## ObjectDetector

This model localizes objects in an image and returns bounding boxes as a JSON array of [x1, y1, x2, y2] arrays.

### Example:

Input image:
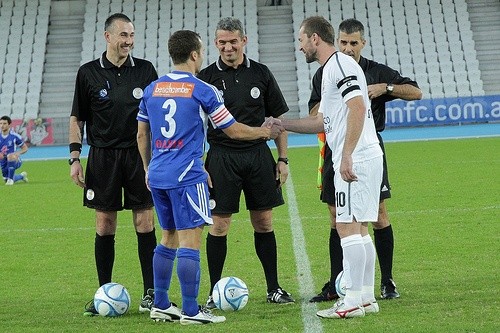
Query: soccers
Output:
[[213, 277, 249, 311], [94, 283, 130, 316]]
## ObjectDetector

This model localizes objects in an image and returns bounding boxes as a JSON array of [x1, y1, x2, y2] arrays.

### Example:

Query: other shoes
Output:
[[20, 171, 28, 183], [5, 177, 14, 185]]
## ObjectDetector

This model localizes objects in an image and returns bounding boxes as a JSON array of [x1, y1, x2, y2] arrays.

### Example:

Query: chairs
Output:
[[0, 0, 51, 119], [80, 0, 260, 78], [292, 0, 485, 118]]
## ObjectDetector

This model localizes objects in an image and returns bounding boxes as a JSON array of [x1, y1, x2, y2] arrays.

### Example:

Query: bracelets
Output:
[[69, 142, 82, 153]]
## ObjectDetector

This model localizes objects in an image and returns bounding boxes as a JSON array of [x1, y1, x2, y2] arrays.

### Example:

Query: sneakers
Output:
[[149, 301, 182, 322], [316, 299, 366, 319], [138, 288, 155, 312], [308, 281, 340, 302], [265, 287, 296, 305], [205, 293, 218, 311], [84, 298, 100, 316], [379, 278, 401, 299], [363, 298, 380, 313], [180, 304, 226, 325]]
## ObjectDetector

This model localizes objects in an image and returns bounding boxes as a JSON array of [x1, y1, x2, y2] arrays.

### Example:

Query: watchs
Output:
[[278, 157, 288, 165], [69, 158, 81, 165], [386, 83, 394, 95]]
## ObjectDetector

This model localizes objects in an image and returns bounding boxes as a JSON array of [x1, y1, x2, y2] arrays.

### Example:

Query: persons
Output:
[[0, 116, 28, 185], [261, 16, 383, 318], [69, 13, 177, 316], [307, 20, 422, 303], [136, 30, 286, 325], [195, 17, 295, 310]]
